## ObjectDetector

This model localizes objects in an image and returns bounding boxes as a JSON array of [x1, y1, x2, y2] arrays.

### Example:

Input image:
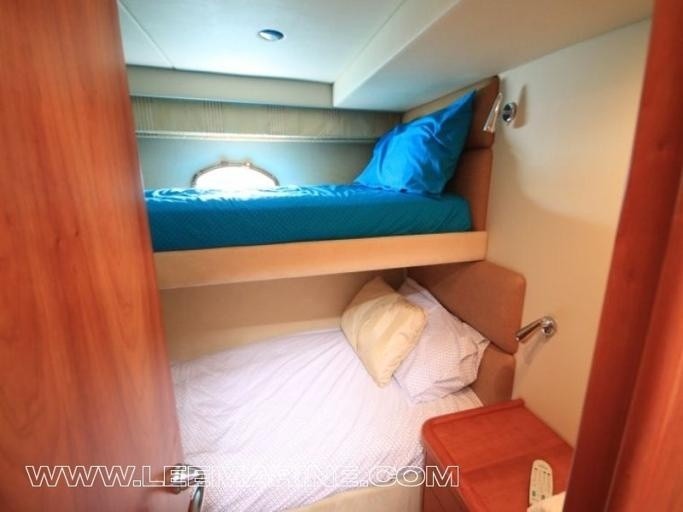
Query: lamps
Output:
[[481, 92, 518, 134]]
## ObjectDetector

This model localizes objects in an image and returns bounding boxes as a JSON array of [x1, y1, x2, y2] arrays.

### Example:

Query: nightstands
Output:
[[419, 398, 576, 512]]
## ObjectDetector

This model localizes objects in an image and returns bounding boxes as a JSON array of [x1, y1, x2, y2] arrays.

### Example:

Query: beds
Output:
[[166, 262, 526, 511], [141, 74, 501, 292]]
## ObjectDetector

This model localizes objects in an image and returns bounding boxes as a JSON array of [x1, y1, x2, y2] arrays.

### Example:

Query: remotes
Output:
[[528, 458, 553, 505]]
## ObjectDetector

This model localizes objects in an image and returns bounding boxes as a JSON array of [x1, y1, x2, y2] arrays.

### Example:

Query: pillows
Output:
[[340, 275, 491, 407], [354, 90, 475, 196]]
